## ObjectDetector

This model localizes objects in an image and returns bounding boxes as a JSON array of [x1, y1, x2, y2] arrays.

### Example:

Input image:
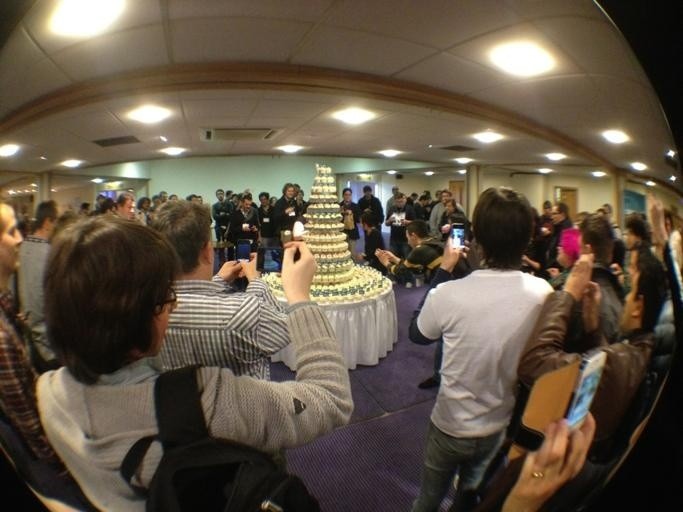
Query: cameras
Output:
[[257, 247, 301, 272]]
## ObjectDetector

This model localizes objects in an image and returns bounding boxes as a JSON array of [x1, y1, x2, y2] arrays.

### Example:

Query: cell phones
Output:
[[400, 218, 404, 222], [236, 239, 251, 263], [562, 350, 607, 432], [451, 223, 465, 249], [249, 223, 254, 229]]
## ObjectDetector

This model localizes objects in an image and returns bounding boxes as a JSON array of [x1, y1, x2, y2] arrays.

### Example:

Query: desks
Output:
[[262, 274, 398, 369]]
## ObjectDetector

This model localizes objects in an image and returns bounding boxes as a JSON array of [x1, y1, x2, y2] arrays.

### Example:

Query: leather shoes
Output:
[[418, 377, 438, 388]]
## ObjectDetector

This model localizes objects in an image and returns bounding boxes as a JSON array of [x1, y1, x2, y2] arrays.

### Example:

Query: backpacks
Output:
[[121, 364, 324, 512]]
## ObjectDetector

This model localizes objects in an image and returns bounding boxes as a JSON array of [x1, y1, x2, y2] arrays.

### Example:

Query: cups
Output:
[[261, 165, 390, 304]]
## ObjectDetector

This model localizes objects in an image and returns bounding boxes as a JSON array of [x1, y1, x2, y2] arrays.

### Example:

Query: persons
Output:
[[3, 181, 683, 512]]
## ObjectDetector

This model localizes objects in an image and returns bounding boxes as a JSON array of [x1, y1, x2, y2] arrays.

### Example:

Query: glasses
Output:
[[153, 287, 177, 315]]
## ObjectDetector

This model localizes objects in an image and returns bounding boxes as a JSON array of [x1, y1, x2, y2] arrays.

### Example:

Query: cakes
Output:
[[258, 162, 391, 306]]
